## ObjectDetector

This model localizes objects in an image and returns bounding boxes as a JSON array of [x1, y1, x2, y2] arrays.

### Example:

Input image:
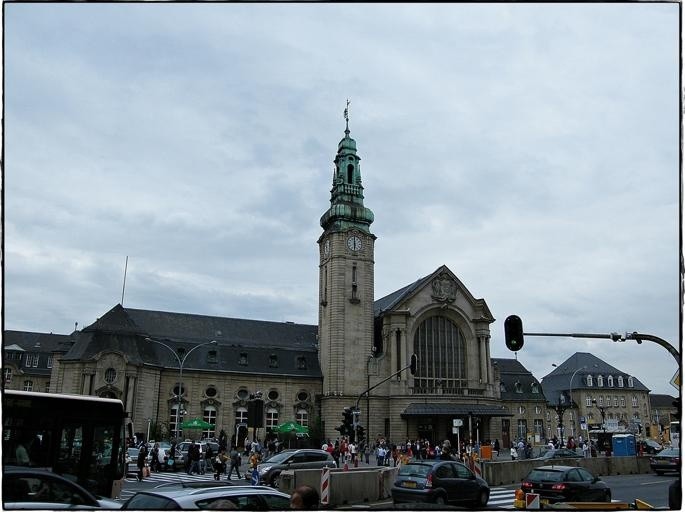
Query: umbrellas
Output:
[[178, 414, 217, 435], [271, 418, 311, 447]]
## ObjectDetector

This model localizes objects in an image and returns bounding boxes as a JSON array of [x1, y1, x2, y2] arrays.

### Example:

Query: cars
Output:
[[521, 465, 610, 505], [245, 450, 338, 486], [390, 459, 490, 509], [650, 448, 681, 476], [126, 441, 220, 480], [635, 437, 662, 454], [122, 481, 290, 510], [3, 468, 123, 509]]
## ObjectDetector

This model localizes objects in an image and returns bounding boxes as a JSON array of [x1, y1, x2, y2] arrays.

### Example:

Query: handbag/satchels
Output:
[[143, 465, 151, 478]]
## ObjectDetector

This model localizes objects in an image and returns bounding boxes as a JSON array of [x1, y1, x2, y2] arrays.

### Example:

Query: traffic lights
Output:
[[335, 407, 367, 444], [410, 354, 417, 374], [463, 414, 482, 429], [504, 315, 523, 351]]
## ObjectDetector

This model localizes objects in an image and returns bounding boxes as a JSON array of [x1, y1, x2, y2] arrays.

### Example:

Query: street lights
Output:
[[145, 336, 218, 441], [552, 364, 588, 438], [247, 391, 264, 443]]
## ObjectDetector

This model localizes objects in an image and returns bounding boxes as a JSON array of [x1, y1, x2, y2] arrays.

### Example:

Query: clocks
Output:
[[323, 239, 331, 256], [346, 233, 363, 252]]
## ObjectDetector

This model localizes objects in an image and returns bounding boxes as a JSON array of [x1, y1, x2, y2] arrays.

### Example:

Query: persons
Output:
[[583, 439, 589, 457], [321, 434, 582, 467], [290, 485, 320, 510], [124, 436, 287, 481], [15, 435, 35, 466]]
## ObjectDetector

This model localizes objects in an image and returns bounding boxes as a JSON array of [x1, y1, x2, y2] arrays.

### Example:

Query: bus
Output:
[[2, 385, 125, 498], [670, 421, 681, 447], [588, 429, 633, 454]]
